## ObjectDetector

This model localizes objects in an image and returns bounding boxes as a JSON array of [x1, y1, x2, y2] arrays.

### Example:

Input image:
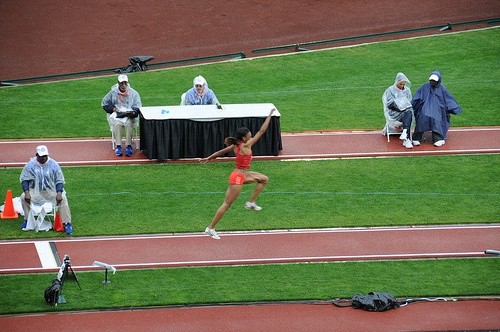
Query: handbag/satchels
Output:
[[44, 278, 60, 306]]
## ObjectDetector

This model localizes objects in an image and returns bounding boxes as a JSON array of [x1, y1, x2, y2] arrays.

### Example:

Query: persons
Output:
[[410, 70, 461, 146], [101, 74, 142, 156], [20, 145, 73, 235], [184, 76, 222, 109], [382, 72, 415, 149], [198, 108, 276, 240]]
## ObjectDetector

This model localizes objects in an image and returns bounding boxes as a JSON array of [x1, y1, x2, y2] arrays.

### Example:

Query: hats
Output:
[[194, 76, 204, 86], [36, 145, 49, 156], [118, 74, 128, 82], [428, 74, 439, 81]]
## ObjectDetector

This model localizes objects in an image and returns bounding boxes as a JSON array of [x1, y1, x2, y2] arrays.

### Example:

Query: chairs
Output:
[[384, 96, 413, 142], [112, 118, 139, 151], [27, 198, 57, 231]]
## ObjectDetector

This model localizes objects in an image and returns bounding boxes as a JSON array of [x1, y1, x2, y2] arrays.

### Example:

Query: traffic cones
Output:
[[0, 189, 18, 219]]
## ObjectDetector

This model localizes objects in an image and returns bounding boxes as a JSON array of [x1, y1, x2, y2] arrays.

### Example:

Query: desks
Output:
[[139, 102, 283, 159]]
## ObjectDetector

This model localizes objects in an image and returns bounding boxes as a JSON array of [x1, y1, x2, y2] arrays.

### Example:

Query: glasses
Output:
[[195, 85, 202, 87], [399, 83, 405, 85]]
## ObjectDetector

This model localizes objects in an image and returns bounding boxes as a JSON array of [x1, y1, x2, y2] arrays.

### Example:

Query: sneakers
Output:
[[399, 134, 407, 140], [403, 140, 413, 148], [21, 220, 27, 229], [64, 222, 73, 235], [412, 140, 421, 146], [114, 146, 123, 156], [205, 227, 220, 240], [125, 146, 133, 157], [433, 139, 445, 147], [245, 201, 262, 211]]
